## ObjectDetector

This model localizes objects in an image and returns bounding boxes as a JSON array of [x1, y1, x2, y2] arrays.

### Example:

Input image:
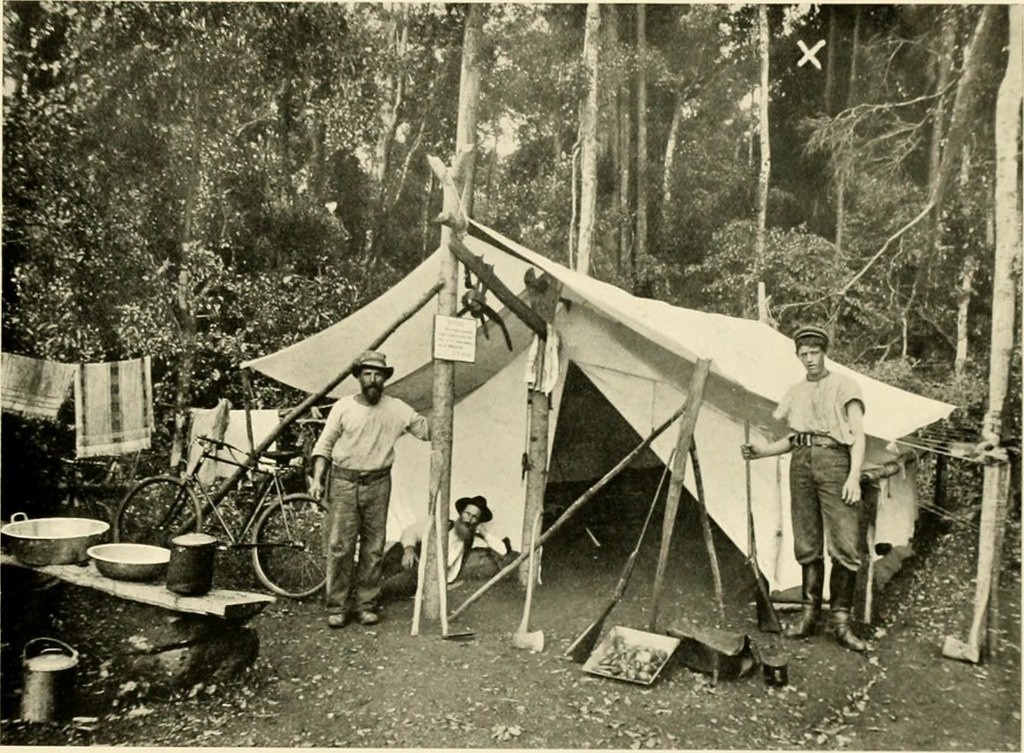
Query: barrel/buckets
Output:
[[21, 636, 80, 722], [166, 532, 218, 597]]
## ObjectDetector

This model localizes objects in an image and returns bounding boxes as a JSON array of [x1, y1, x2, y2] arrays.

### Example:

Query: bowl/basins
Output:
[[0, 511, 111, 566], [84, 541, 172, 583]]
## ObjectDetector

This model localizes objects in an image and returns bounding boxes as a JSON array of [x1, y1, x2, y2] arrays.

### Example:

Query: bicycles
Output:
[[112, 433, 343, 601]]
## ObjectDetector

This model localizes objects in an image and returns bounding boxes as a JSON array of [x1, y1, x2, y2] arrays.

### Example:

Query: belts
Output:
[[332, 469, 392, 483], [792, 433, 835, 446]]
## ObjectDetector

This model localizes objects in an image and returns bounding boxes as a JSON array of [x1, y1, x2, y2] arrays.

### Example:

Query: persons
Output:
[[741, 325, 870, 651], [308, 351, 432, 626], [375, 496, 510, 596]]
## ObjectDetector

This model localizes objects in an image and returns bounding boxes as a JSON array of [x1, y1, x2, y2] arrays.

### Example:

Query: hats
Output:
[[351, 351, 397, 378], [453, 495, 493, 524], [793, 324, 829, 341]]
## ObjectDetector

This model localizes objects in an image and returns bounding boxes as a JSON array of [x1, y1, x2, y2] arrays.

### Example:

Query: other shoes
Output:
[[355, 611, 379, 623], [328, 611, 349, 628]]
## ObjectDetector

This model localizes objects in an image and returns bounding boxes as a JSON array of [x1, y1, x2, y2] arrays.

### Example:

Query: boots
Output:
[[789, 565, 824, 637], [827, 565, 866, 654]]
